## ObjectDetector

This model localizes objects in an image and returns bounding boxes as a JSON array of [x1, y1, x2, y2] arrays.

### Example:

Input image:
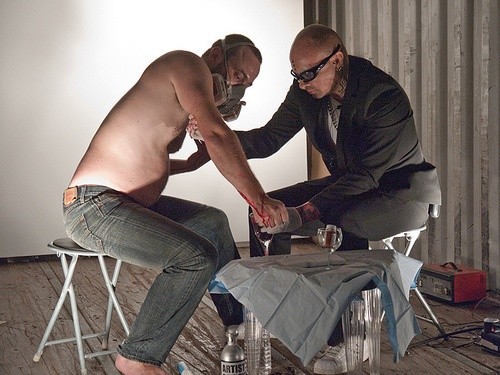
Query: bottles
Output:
[[220, 330, 247, 375]]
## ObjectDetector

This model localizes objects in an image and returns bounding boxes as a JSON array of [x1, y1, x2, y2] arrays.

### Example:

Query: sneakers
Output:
[[237, 322, 278, 339], [314, 332, 372, 374]]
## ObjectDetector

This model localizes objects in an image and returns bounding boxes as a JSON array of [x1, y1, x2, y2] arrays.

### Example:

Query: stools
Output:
[[368, 225, 450, 355], [32, 238, 131, 375]]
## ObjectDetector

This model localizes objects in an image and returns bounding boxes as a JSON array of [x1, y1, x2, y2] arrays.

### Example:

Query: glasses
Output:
[[290, 44, 340, 82]]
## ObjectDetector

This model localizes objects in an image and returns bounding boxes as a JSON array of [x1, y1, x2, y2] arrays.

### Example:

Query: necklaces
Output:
[[326, 96, 338, 130]]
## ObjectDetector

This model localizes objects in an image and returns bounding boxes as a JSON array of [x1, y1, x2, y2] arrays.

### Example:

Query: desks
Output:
[[214, 245, 423, 374]]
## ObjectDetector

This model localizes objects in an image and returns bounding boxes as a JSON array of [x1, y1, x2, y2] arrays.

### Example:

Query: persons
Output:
[[63, 34, 289, 375], [187, 25, 441, 375]]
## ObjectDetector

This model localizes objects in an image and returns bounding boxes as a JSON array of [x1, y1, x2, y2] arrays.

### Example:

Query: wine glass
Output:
[[249, 213, 277, 268], [317, 227, 343, 270]]
